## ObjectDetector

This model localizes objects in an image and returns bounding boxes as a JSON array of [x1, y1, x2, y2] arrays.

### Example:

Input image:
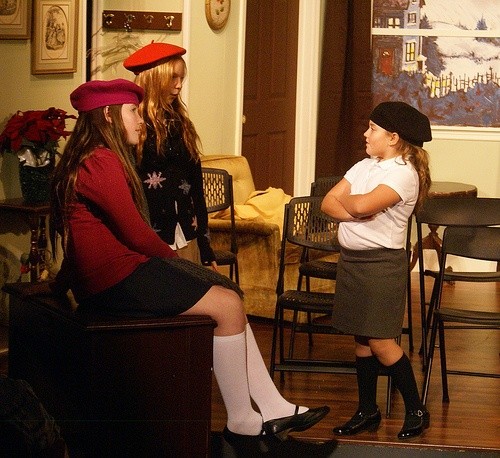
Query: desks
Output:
[[411, 182, 478, 286]]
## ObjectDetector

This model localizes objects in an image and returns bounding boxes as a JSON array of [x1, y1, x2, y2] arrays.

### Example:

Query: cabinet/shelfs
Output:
[[1, 283, 216, 458], [0, 199, 60, 283]]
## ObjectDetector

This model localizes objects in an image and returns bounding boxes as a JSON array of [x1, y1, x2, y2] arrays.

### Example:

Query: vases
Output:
[[20, 166, 56, 207]]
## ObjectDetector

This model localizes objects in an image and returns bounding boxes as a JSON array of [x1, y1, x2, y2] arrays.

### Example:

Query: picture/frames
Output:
[[0, 0, 32, 40], [31, 0, 80, 75]]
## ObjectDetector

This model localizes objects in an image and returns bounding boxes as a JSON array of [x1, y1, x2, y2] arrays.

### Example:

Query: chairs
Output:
[[270, 174, 500, 419]]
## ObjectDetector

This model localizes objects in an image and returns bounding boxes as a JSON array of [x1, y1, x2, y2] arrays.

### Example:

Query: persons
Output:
[[123, 41, 220, 271], [49, 79, 338, 458], [321, 101, 432, 441]]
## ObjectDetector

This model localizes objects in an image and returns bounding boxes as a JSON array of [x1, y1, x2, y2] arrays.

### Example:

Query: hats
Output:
[[70, 79, 144, 115], [123, 40, 186, 75], [370, 102, 432, 147]]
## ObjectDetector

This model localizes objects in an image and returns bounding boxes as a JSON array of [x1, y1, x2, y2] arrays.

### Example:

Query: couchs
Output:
[[198, 154, 342, 325]]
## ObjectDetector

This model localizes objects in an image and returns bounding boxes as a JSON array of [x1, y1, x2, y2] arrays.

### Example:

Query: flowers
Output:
[[0, 108, 75, 164]]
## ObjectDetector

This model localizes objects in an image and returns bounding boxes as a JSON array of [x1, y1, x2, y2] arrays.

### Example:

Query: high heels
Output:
[[263, 405, 330, 440], [224, 425, 294, 458], [398, 407, 430, 439], [333, 408, 381, 435]]
